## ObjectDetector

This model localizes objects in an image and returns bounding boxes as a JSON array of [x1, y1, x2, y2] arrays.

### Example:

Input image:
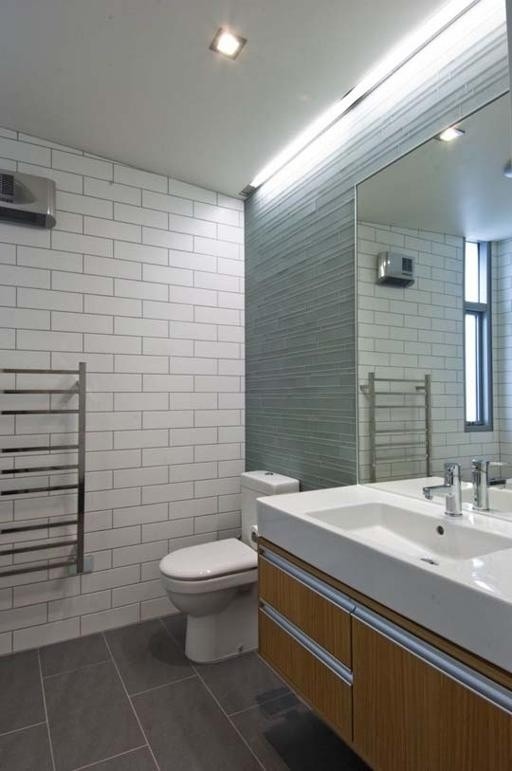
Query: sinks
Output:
[[456, 553, 507, 604], [309, 501, 512, 566], [460, 486, 511, 518]]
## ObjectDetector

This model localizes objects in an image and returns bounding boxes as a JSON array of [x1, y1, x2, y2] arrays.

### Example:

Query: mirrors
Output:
[[350, 92, 512, 522]]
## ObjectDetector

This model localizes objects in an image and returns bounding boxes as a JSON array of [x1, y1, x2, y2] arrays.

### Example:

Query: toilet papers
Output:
[[248, 525, 258, 549]]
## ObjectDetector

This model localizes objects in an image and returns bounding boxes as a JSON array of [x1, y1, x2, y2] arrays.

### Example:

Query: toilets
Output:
[[158, 467, 301, 664]]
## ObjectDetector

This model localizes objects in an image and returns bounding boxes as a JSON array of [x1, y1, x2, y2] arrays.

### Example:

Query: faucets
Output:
[[472, 456, 511, 509], [422, 463, 464, 518]]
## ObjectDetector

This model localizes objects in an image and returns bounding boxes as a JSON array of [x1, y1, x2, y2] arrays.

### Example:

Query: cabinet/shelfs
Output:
[[259, 540, 512, 771]]
